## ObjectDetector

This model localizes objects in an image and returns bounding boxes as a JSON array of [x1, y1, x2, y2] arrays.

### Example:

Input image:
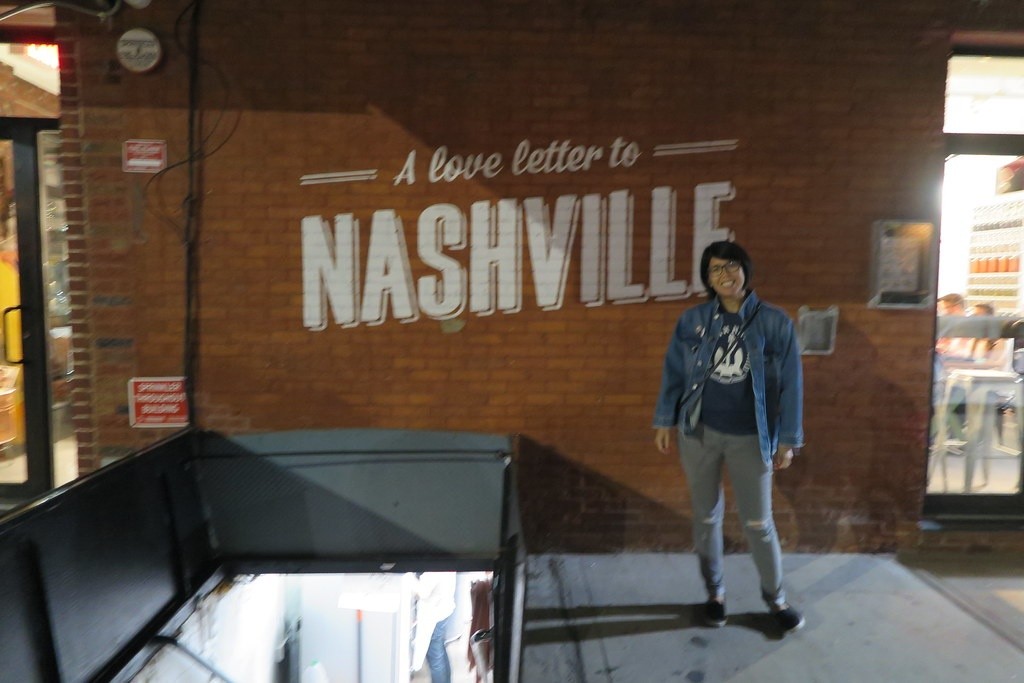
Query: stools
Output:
[[928, 370, 1022, 493]]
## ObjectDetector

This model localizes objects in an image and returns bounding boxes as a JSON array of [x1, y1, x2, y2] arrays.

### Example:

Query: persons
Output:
[[932, 294, 1005, 436], [412, 570, 456, 683], [652, 240, 807, 632]]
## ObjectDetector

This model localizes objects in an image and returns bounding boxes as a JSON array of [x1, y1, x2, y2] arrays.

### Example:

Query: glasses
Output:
[[704, 259, 744, 277]]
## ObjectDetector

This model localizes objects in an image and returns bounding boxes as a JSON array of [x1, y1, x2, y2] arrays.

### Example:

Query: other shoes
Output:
[[770, 602, 805, 633], [705, 600, 728, 627]]
